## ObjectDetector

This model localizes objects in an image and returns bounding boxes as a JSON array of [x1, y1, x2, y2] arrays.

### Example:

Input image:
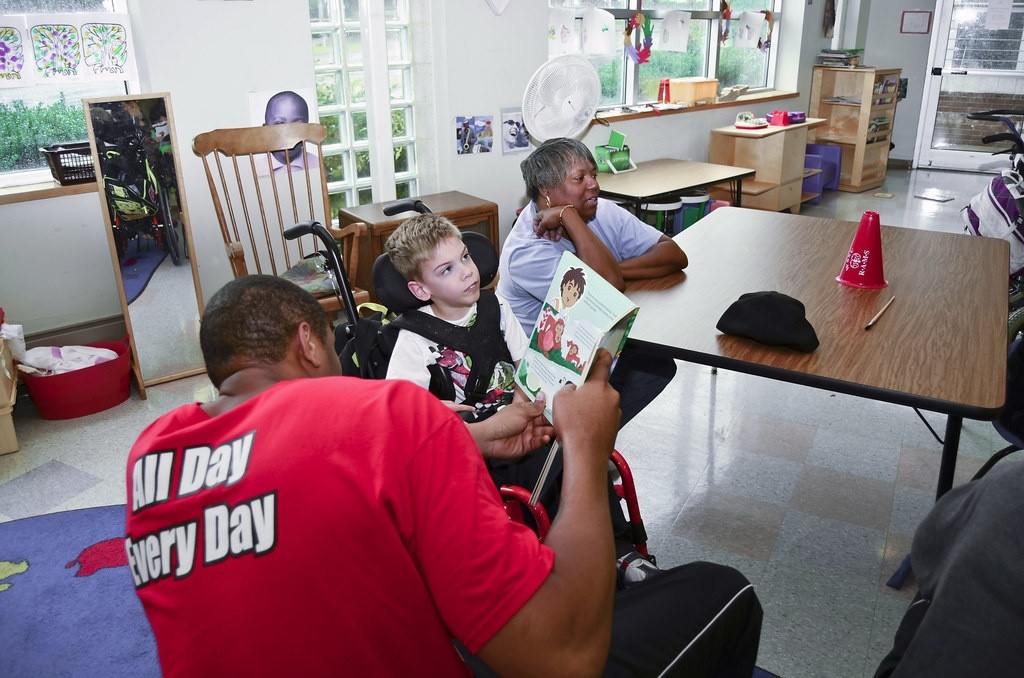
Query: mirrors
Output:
[[82, 92, 206, 386]]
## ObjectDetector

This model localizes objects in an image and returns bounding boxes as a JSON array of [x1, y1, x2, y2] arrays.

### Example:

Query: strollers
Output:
[[90, 108, 182, 267], [280, 198, 656, 591], [966, 108, 1024, 452]]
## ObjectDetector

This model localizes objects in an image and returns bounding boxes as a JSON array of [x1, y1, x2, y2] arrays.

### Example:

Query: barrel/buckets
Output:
[[13, 333, 131, 420]]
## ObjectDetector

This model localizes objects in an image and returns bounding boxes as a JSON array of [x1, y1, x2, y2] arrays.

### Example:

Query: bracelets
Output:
[[559, 204, 574, 226]]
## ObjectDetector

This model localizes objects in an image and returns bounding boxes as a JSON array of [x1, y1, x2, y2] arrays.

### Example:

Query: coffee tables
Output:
[[339, 191, 499, 260]]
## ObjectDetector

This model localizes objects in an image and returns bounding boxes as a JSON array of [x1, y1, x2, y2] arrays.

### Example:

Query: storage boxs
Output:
[[670, 77, 719, 102], [41, 141, 96, 186]]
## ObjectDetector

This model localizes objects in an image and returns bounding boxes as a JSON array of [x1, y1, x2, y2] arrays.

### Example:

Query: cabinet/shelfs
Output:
[[709, 118, 828, 216], [809, 67, 902, 192]]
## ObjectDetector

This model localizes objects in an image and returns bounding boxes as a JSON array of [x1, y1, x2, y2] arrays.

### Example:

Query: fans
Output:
[[522, 55, 601, 141]]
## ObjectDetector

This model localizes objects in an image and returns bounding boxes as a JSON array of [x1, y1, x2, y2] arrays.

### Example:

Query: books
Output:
[[514, 251, 641, 508]]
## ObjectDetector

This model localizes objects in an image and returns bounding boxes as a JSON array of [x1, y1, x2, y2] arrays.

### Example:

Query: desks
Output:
[[597, 160, 1010, 502]]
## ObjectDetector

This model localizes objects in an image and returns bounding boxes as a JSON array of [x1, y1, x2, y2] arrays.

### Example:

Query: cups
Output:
[[657, 78, 670, 103]]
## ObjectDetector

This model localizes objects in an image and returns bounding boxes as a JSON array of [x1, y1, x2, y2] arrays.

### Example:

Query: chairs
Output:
[[193, 123, 369, 332]]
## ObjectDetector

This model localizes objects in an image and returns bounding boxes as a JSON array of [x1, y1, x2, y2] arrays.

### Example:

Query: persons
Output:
[[257, 91, 318, 176], [502, 114, 529, 151], [461, 120, 477, 153], [386, 212, 658, 581], [497, 138, 688, 430], [125, 274, 622, 678]]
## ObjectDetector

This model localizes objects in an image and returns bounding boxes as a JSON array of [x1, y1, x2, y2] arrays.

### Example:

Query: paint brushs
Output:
[[864, 296, 896, 330]]
